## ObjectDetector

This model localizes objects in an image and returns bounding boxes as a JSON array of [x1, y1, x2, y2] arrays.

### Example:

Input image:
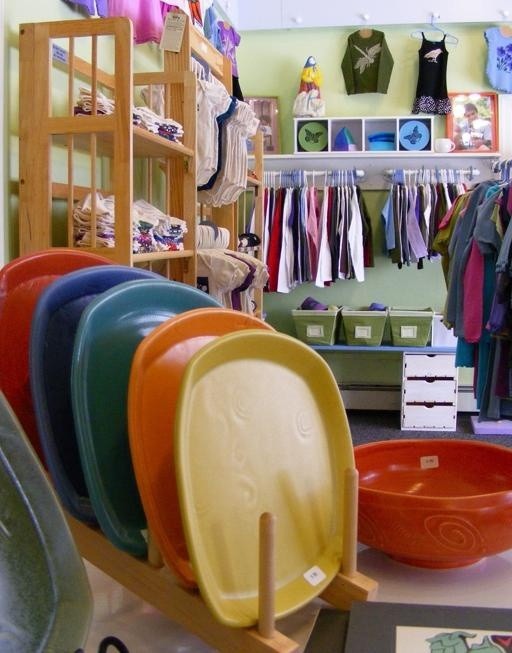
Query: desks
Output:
[[308, 346, 460, 432]]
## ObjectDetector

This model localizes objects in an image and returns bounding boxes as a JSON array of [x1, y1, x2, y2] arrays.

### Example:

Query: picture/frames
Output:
[[243, 96, 280, 155], [446, 93, 497, 153]]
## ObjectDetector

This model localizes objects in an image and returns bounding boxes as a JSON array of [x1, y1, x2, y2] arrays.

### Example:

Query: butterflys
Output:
[[303, 128, 323, 145], [404, 124, 423, 146]]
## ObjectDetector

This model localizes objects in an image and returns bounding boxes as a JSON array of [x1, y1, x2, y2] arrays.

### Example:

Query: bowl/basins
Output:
[[341, 437, 512, 569]]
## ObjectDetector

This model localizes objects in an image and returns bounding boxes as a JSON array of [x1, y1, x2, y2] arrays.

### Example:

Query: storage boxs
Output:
[[341, 305, 389, 347], [431, 311, 457, 348], [389, 305, 435, 347], [292, 305, 343, 346]]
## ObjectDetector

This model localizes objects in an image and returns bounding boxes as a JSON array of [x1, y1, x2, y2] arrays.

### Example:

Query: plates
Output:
[[0, 245, 361, 652]]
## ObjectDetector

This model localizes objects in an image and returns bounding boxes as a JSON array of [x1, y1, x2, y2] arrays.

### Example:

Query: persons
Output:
[[454, 103, 491, 149], [470, 132, 490, 151]]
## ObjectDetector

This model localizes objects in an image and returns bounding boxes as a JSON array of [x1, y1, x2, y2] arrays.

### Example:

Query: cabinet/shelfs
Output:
[[293, 116, 435, 153], [19, 12, 262, 323]]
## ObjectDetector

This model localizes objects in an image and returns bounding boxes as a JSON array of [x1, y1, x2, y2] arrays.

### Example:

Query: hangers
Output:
[[262, 168, 357, 188], [197, 223, 231, 252], [358, 18, 372, 38], [499, 14, 512, 38], [192, 58, 219, 85], [490, 158, 512, 183], [385, 165, 474, 188], [410, 14, 459, 46]]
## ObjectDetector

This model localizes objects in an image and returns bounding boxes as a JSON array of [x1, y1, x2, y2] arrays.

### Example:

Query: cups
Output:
[[434, 138, 456, 152]]
